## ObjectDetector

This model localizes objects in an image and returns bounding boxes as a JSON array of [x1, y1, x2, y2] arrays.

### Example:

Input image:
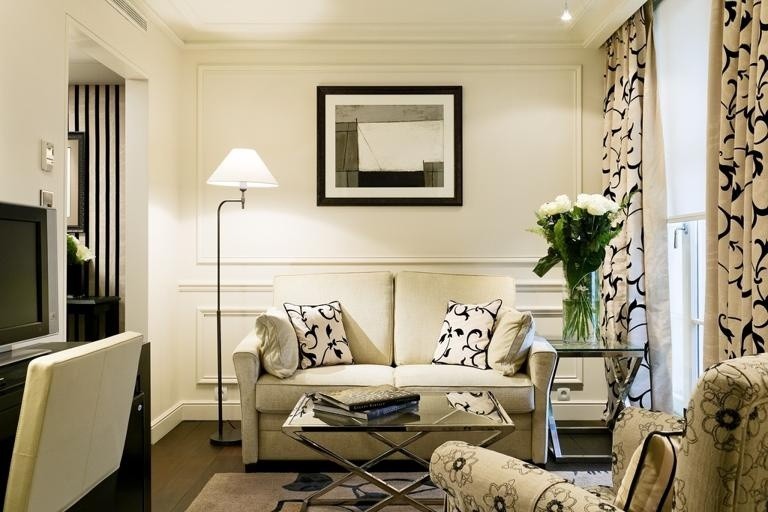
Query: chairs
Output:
[[427, 350, 767, 511]]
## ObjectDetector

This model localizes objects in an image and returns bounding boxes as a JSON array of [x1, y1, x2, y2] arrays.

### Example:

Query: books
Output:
[[313, 401, 419, 420], [319, 384, 420, 412]]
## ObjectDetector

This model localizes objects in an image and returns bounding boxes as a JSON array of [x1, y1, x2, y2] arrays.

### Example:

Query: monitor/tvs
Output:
[[0, 201, 58, 346]]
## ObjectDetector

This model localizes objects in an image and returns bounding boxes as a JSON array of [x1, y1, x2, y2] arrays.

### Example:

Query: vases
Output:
[[68, 258, 90, 295], [563, 266, 604, 347]]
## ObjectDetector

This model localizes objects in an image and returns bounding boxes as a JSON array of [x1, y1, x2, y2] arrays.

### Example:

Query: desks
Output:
[[66, 295, 121, 343], [543, 338, 650, 468]]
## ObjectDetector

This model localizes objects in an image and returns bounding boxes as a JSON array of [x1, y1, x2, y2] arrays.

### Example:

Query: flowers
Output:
[[526, 192, 627, 337], [67, 238, 94, 267]]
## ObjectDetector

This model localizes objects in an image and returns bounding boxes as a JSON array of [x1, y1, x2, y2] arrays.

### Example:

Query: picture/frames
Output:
[[316, 82, 465, 210], [64, 132, 88, 234]]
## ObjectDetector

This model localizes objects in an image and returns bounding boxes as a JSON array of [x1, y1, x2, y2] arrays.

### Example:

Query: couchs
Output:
[[231, 269, 551, 472]]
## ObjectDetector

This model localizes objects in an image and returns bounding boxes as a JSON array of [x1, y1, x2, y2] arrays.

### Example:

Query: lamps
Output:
[[206, 145, 279, 447]]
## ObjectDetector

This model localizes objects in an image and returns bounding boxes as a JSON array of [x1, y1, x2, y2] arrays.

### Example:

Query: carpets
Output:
[[178, 466, 619, 511]]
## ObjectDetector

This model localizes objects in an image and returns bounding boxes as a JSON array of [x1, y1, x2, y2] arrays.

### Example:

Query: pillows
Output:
[[284, 302, 354, 368], [488, 302, 532, 377], [617, 431, 681, 511], [431, 298, 505, 372], [251, 309, 300, 381]]
